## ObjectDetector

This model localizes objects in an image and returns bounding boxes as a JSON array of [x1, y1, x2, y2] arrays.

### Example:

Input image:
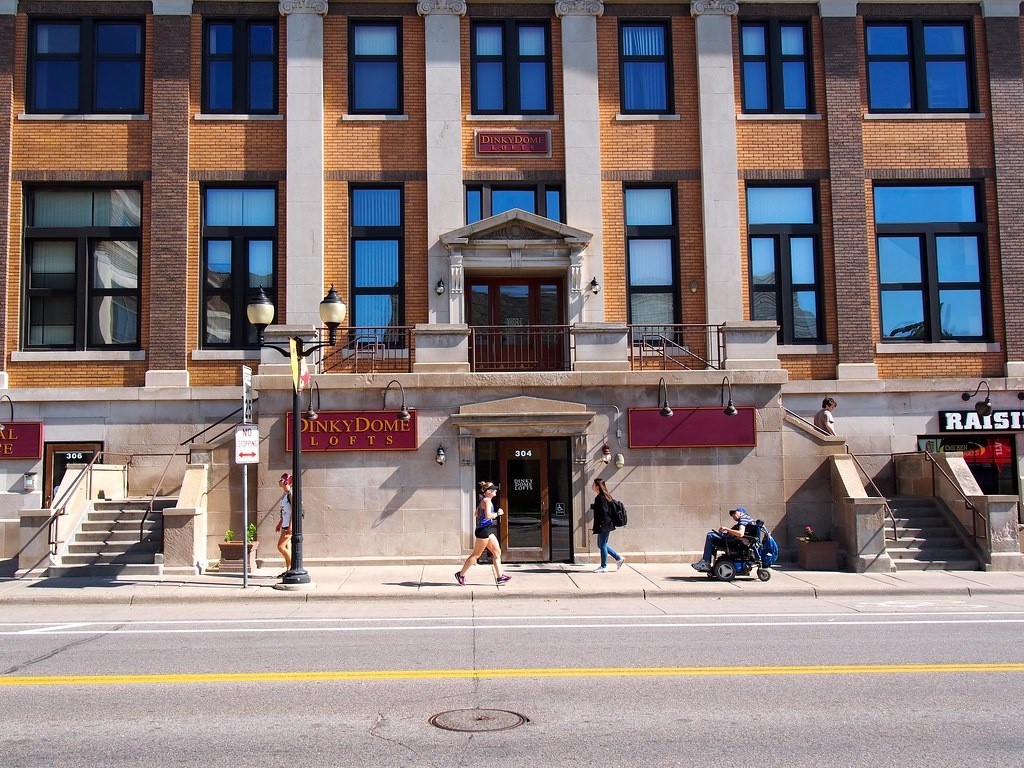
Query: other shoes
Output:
[[593, 566, 607, 573], [455, 571, 465, 586], [616, 557, 625, 570], [497, 574, 512, 584]]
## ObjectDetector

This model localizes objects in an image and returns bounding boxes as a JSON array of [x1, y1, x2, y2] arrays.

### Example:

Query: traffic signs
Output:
[[235, 424, 262, 464]]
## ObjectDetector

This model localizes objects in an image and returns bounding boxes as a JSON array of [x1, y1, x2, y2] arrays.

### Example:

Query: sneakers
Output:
[[691, 559, 711, 570]]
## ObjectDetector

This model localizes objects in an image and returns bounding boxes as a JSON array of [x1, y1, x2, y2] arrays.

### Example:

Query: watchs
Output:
[[497, 512, 500, 516]]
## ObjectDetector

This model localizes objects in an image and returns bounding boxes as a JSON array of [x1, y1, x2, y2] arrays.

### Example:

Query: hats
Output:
[[483, 484, 499, 493], [281, 472, 292, 485], [729, 507, 747, 517]]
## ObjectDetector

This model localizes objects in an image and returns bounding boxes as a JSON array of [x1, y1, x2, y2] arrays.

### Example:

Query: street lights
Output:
[[247, 282, 348, 584]]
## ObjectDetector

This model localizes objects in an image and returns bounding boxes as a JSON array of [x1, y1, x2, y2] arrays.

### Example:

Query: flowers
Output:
[[803, 526, 834, 542]]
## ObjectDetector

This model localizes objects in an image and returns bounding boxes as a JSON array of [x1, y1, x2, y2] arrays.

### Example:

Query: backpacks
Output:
[[757, 526, 778, 568], [609, 499, 627, 527]]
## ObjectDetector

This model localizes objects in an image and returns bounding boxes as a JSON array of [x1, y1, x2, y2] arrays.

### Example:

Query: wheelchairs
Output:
[[701, 520, 771, 582]]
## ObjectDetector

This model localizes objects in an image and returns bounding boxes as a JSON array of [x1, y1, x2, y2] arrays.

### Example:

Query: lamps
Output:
[[615, 454, 624, 469], [658, 378, 674, 418], [963, 380, 992, 416], [601, 443, 612, 465], [590, 277, 601, 295], [304, 380, 320, 421], [435, 277, 445, 297], [0, 395, 14, 431], [435, 444, 447, 465], [721, 376, 738, 417], [383, 380, 411, 421]]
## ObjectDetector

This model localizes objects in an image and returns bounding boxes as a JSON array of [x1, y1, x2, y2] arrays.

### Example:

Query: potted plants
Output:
[[218, 523, 259, 560]]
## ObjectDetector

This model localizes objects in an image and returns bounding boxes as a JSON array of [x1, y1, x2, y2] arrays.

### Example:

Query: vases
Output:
[[796, 536, 840, 569]]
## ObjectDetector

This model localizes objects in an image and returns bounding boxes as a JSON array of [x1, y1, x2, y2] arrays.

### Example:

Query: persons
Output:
[[814, 398, 837, 437], [275, 473, 292, 579], [691, 508, 756, 571], [592, 478, 625, 572], [455, 482, 512, 586]]
